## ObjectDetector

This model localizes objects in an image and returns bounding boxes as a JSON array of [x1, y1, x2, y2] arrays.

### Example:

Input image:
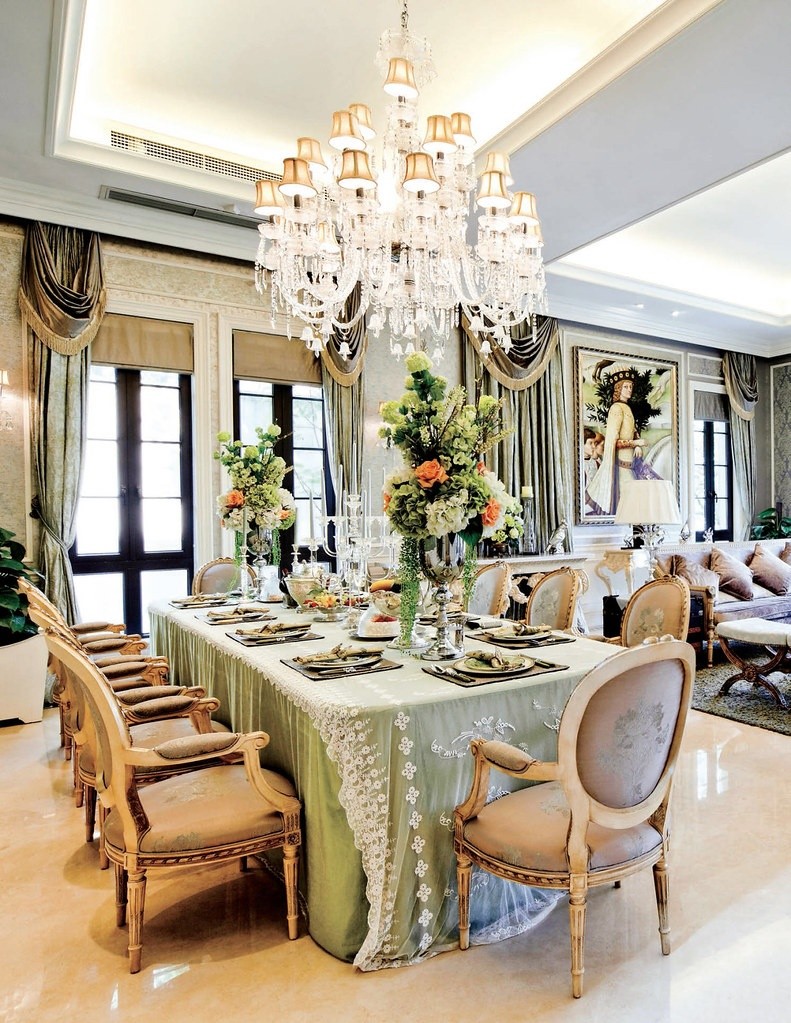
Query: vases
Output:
[[246, 527, 272, 601], [490, 541, 512, 558], [418, 532, 467, 660]]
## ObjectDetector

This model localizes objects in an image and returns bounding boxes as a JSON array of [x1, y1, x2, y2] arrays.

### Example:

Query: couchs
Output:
[[641, 538, 791, 668]]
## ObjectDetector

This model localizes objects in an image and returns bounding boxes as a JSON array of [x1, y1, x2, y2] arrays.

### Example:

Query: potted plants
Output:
[[0, 527, 50, 724]]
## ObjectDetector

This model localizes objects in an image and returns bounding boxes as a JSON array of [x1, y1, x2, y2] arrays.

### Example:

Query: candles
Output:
[[521, 485, 534, 497], [368, 469, 372, 516], [361, 490, 368, 538], [343, 489, 349, 537], [241, 506, 248, 552], [336, 464, 342, 515], [308, 489, 315, 540], [293, 507, 299, 546], [352, 443, 359, 495], [381, 467, 386, 516], [321, 470, 326, 517]]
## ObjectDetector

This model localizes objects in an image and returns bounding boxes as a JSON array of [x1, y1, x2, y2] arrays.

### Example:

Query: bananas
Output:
[[369, 580, 394, 592]]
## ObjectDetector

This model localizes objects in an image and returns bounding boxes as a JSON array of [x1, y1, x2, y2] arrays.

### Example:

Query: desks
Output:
[[464, 552, 589, 615]]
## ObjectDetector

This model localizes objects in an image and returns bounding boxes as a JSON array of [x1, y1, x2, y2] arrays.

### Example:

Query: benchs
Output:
[[715, 617, 791, 710]]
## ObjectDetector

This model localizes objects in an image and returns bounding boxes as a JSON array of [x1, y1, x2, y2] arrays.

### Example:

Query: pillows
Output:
[[671, 554, 720, 604], [710, 546, 754, 602], [780, 542, 791, 566], [749, 543, 791, 595]]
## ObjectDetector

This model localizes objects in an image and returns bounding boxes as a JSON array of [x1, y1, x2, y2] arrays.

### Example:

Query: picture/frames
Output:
[[571, 343, 690, 528]]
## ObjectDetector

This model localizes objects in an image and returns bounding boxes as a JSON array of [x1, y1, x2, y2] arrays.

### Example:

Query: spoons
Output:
[[430, 663, 477, 683]]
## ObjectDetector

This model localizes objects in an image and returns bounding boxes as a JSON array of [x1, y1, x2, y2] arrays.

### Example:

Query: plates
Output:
[[483, 627, 552, 640], [239, 629, 309, 639], [453, 654, 535, 674], [301, 652, 382, 669]]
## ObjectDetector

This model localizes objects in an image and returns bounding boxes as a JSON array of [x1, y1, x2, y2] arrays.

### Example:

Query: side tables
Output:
[[594, 548, 651, 596], [601, 594, 706, 672]]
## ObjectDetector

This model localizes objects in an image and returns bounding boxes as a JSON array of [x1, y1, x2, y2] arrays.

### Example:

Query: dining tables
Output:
[[148, 588, 631, 967]]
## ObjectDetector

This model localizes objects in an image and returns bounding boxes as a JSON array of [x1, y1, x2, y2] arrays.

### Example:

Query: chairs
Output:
[[463, 561, 509, 617], [27, 589, 168, 809], [42, 627, 302, 973], [12, 576, 142, 759], [525, 566, 580, 631], [191, 556, 258, 596], [27, 603, 244, 870], [453, 633, 697, 1002], [605, 575, 691, 649]]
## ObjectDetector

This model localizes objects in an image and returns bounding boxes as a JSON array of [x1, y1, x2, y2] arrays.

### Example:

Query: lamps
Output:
[[250, 0, 552, 364], [612, 480, 682, 547]]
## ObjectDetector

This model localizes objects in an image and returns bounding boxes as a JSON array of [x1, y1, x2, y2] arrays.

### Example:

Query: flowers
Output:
[[213, 416, 298, 601], [379, 353, 527, 653]]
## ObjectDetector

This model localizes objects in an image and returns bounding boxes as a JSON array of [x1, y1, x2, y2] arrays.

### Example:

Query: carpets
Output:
[[690, 639, 791, 736]]
